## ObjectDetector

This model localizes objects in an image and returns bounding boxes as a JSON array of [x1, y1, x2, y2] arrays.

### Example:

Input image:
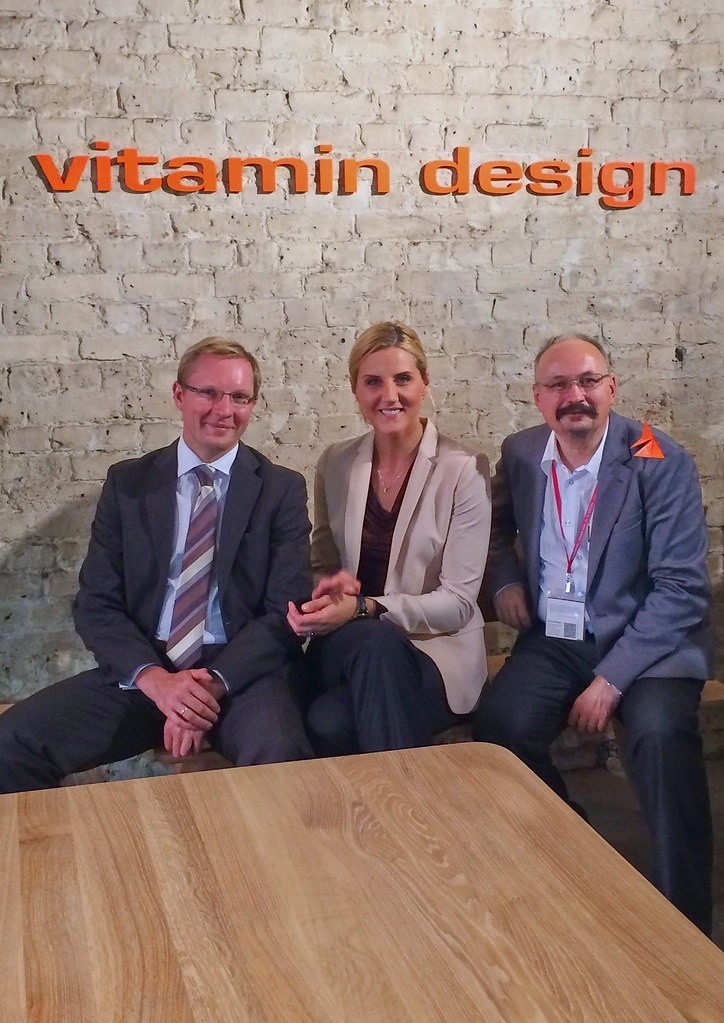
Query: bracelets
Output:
[[605, 682, 625, 699]]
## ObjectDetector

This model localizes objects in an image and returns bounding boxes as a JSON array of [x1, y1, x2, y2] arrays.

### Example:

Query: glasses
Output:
[[537, 373, 610, 392], [181, 384, 258, 404]]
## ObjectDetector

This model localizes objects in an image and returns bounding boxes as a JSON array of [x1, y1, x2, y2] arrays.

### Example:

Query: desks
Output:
[[0, 741, 724, 1023]]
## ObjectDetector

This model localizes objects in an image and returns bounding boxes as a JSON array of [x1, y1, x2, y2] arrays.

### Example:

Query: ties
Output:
[[167, 467, 217, 671]]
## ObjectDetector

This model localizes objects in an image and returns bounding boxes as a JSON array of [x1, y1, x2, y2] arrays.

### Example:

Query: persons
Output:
[[0, 335, 312, 797], [286, 318, 493, 755], [473, 330, 714, 945]]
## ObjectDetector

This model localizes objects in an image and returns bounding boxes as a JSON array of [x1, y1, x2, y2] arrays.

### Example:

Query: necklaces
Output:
[[376, 467, 403, 494]]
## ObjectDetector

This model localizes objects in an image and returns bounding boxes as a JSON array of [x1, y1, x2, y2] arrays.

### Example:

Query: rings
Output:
[[311, 632, 314, 636], [180, 707, 189, 717]]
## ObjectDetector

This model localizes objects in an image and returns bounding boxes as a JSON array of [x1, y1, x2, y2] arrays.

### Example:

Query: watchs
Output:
[[349, 594, 369, 621]]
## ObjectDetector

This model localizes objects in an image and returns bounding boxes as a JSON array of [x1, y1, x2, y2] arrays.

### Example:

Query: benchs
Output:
[[154, 655, 723, 773]]
